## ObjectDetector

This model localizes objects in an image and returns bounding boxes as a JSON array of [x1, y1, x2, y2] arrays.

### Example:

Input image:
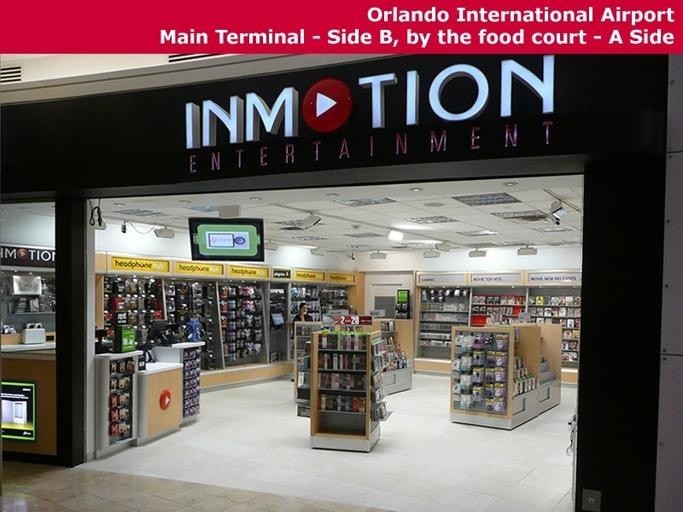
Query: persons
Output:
[[292, 302, 313, 381]]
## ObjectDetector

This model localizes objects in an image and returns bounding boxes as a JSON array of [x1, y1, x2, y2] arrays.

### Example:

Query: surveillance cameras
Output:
[[121, 224, 128, 235], [549, 213, 561, 225]]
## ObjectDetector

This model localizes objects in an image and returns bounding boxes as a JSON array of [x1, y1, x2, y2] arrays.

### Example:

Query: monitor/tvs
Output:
[[149, 318, 171, 344], [189, 218, 265, 261]]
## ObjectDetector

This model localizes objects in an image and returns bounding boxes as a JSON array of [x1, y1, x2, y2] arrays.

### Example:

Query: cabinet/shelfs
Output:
[[420, 293, 581, 430], [293, 319, 412, 452]]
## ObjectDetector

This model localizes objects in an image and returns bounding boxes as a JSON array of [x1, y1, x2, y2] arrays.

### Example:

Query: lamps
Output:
[[153, 226, 175, 240], [422, 239, 539, 259], [369, 248, 387, 260], [265, 238, 279, 250], [310, 245, 327, 256]]
